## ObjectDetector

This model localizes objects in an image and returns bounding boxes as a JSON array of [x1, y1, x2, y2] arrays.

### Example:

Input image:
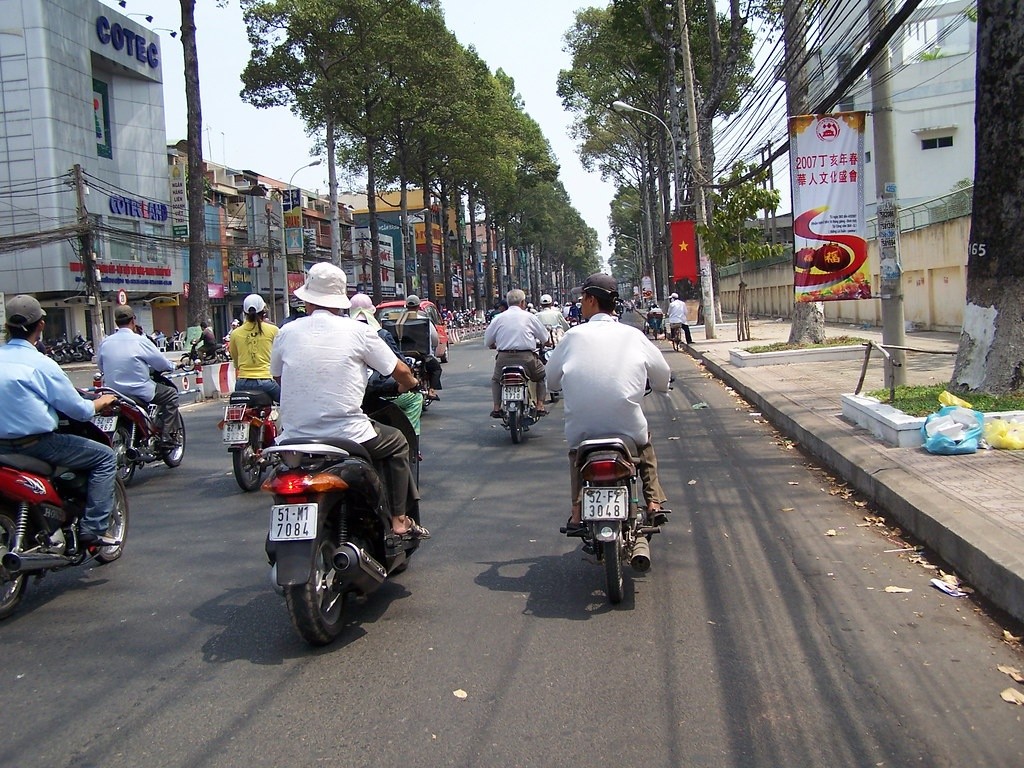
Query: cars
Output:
[[374, 299, 450, 364]]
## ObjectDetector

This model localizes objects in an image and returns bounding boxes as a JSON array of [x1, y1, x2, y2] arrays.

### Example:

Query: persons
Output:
[[0, 294, 123, 548], [226, 320, 241, 339], [405, 296, 442, 402], [97, 305, 183, 448], [536, 295, 570, 331], [526, 303, 538, 314], [490, 300, 508, 323], [611, 310, 620, 322], [553, 301, 589, 326], [229, 294, 282, 404], [134, 325, 180, 351], [343, 295, 425, 462], [667, 294, 695, 343], [544, 272, 671, 534], [271, 261, 430, 540], [649, 303, 663, 314], [484, 289, 550, 417], [196, 321, 216, 365], [441, 307, 476, 329]]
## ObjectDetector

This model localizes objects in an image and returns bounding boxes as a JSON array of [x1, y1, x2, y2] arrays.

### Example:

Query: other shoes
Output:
[[671, 338, 675, 341], [160, 438, 182, 448], [687, 341, 695, 344], [77, 533, 121, 546], [428, 393, 440, 400], [490, 411, 501, 417], [200, 360, 206, 365], [418, 451, 423, 461], [537, 410, 549, 416]]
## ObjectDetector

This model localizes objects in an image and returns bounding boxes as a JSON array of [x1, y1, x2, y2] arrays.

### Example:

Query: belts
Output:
[[0, 435, 44, 445], [498, 350, 531, 352]]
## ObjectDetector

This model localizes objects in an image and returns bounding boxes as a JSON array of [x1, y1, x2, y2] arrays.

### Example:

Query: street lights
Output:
[[610, 100, 717, 340], [279, 157, 324, 318], [400, 206, 430, 300], [620, 200, 658, 304], [620, 233, 648, 281]]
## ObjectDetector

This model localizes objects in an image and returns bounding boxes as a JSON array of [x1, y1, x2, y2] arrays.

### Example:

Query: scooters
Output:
[[34, 331, 95, 365], [534, 301, 633, 404]]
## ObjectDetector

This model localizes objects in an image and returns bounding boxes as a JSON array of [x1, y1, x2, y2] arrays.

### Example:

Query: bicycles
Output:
[[643, 311, 664, 340], [665, 315, 681, 352]]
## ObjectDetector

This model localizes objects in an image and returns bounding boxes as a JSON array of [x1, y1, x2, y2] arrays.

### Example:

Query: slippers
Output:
[[646, 505, 668, 526], [567, 515, 582, 530], [390, 518, 431, 540]]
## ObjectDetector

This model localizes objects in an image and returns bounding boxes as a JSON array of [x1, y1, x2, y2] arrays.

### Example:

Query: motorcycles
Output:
[[179, 339, 230, 373], [399, 343, 439, 412], [261, 370, 422, 647], [544, 374, 676, 605], [489, 341, 554, 445], [218, 391, 283, 493], [1, 386, 131, 622], [94, 363, 187, 487]]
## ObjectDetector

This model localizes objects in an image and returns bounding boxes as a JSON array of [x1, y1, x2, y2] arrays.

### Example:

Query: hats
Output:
[[406, 295, 420, 306], [668, 293, 679, 298], [293, 261, 352, 309], [243, 293, 266, 314], [349, 294, 382, 331], [527, 302, 533, 308], [234, 319, 240, 324], [231, 322, 238, 326], [572, 272, 624, 304], [5, 295, 47, 331], [114, 304, 136, 321], [539, 294, 553, 304]]
